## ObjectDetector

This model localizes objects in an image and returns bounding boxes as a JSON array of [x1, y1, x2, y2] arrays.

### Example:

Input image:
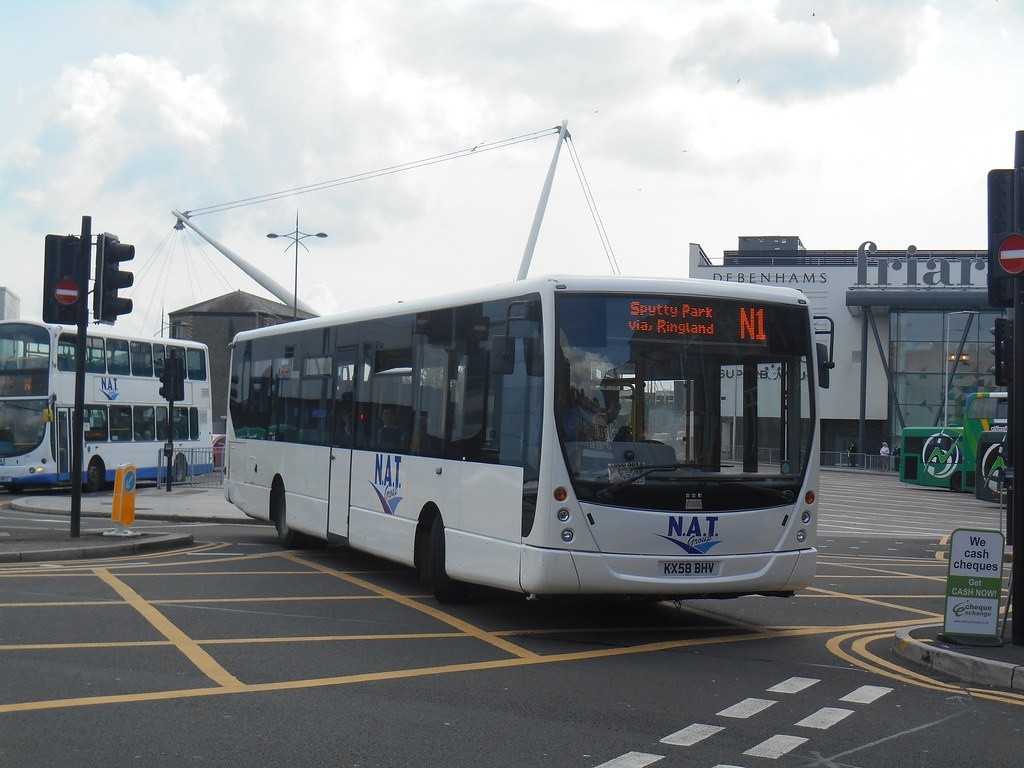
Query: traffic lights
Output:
[[94, 231, 136, 326]]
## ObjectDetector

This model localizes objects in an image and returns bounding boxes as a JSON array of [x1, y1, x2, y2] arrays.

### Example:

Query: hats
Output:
[[882, 442, 888, 446]]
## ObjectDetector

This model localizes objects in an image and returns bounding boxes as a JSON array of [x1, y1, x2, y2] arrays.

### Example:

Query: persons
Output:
[[260, 359, 621, 479], [848, 441, 856, 467], [891, 445, 900, 472], [880, 442, 890, 473]]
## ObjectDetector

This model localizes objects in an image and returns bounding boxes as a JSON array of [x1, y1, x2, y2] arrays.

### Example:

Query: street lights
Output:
[[945, 310, 981, 426], [267, 231, 327, 322]]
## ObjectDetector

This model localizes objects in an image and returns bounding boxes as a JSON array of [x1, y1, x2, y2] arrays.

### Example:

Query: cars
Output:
[[212, 434, 226, 468]]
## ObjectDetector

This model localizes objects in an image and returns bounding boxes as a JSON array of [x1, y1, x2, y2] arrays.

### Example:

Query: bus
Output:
[[899, 427, 963, 493], [962, 391, 1007, 493], [0, 318, 215, 491], [221, 275, 835, 604]]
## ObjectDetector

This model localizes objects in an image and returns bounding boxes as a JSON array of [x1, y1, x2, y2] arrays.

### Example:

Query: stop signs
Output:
[[998, 233, 1024, 274], [54, 280, 80, 305]]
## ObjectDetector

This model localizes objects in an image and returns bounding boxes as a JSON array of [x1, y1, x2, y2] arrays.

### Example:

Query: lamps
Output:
[[947, 353, 971, 366]]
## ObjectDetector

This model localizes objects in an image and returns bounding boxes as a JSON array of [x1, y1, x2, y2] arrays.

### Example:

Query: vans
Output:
[[977, 430, 1007, 502]]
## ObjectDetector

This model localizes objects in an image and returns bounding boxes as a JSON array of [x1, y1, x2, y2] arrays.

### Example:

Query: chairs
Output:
[[5, 354, 157, 378]]
